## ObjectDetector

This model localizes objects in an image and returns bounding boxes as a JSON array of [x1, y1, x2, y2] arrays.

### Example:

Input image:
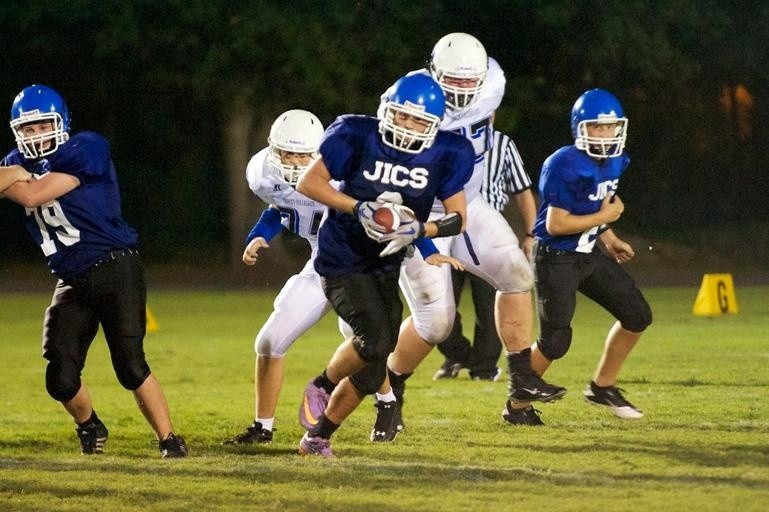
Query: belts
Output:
[[90, 249, 139, 269]]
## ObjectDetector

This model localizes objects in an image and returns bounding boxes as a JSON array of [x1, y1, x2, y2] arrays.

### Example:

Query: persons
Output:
[[296, 73, 475, 455], [375, 31, 565, 428], [0, 165, 31, 192], [221, 108, 400, 444], [0, 83, 188, 460], [531, 88, 653, 421]]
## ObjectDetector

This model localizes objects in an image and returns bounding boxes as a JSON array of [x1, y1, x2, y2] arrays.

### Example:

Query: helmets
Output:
[[571, 88, 629, 158], [378, 73, 445, 155], [10, 85, 71, 158], [266, 109, 324, 186], [426, 32, 488, 111]]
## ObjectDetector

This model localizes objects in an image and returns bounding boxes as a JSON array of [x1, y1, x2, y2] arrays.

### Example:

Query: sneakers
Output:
[[299, 368, 414, 459], [221, 420, 277, 446], [582, 381, 644, 419], [471, 365, 501, 382], [433, 357, 468, 379], [151, 433, 188, 458], [76, 421, 108, 454], [503, 380, 566, 425]]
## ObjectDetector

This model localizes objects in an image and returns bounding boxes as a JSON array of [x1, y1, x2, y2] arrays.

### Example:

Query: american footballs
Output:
[[372, 203, 417, 237]]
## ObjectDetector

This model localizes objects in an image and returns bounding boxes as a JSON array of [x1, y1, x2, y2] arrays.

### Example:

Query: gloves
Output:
[[377, 203, 426, 257], [352, 201, 394, 241]]
